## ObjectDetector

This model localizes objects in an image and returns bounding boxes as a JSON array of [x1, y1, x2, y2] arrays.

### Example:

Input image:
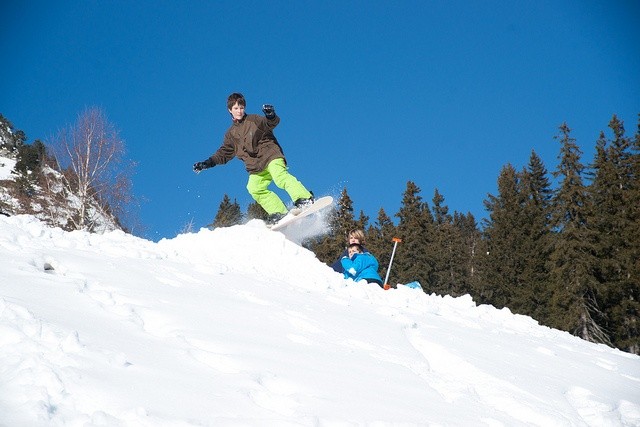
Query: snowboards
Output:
[[268, 196, 334, 231]]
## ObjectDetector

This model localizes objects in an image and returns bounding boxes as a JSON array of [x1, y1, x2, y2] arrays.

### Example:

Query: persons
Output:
[[193, 94, 316, 228], [326, 230, 383, 287], [341, 242, 382, 287]]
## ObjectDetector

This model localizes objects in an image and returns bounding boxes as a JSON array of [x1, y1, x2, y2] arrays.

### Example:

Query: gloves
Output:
[[261, 103, 274, 117], [193, 157, 215, 172]]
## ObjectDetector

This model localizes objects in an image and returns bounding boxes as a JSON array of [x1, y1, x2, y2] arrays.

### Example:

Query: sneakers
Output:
[[265, 214, 285, 227], [290, 196, 313, 216]]
